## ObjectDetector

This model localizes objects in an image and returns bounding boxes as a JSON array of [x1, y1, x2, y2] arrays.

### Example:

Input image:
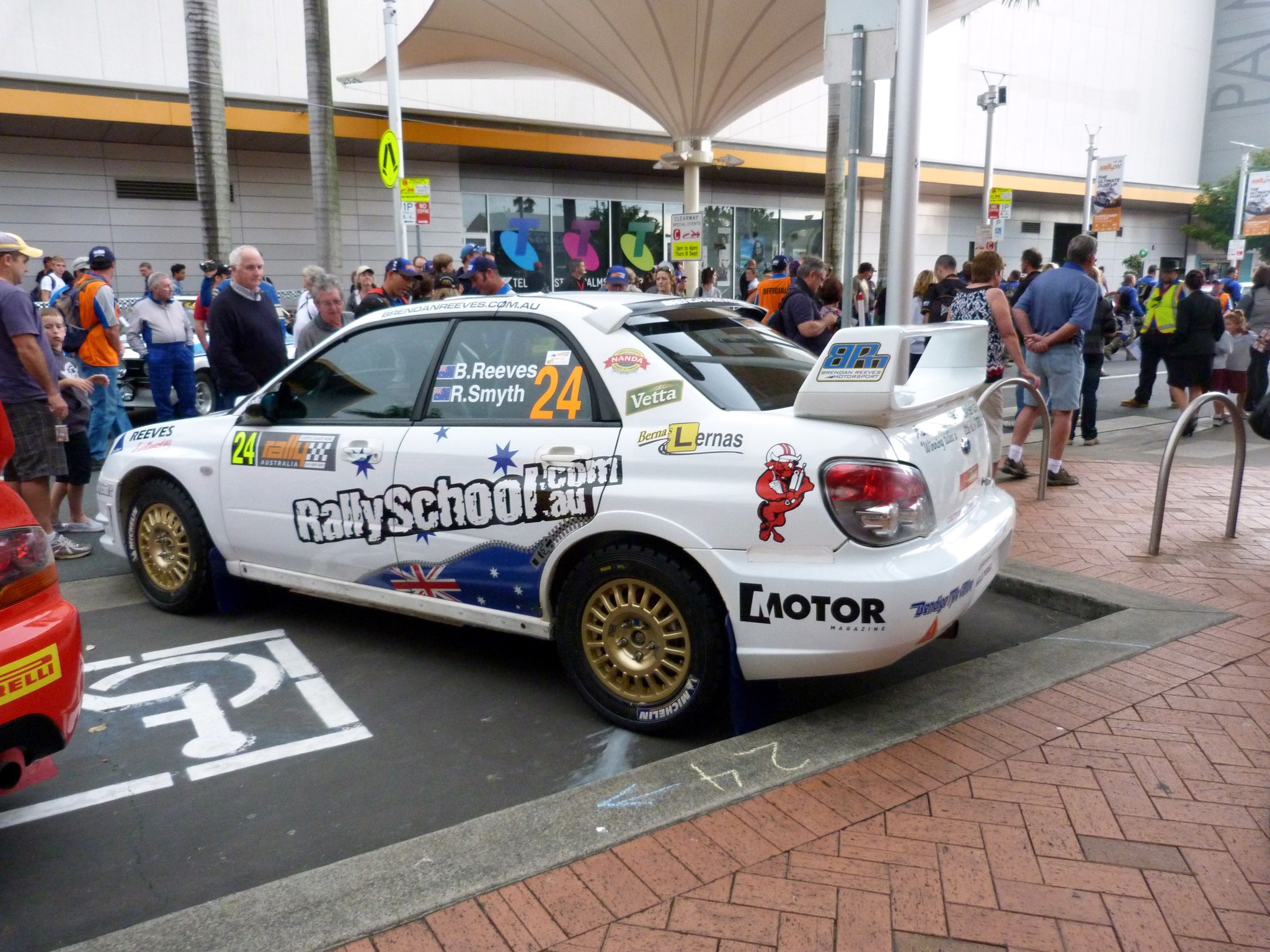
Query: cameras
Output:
[[200, 260, 222, 272], [55, 425, 69, 442], [832, 312, 842, 327]]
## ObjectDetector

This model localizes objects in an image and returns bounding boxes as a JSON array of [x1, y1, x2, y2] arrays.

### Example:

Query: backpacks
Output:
[[51, 276, 118, 353], [930, 281, 955, 322]]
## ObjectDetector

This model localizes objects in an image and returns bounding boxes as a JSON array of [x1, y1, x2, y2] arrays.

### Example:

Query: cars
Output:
[[107, 293, 298, 419], [92, 288, 1024, 747]]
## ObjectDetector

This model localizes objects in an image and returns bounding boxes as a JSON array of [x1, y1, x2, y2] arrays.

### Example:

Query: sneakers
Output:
[[1212, 414, 1233, 426], [68, 517, 106, 532], [1066, 440, 1073, 444], [50, 523, 69, 532], [1047, 468, 1079, 486], [1171, 402, 1179, 408], [1002, 457, 1029, 478], [48, 532, 93, 559], [1120, 398, 1148, 407], [1240, 409, 1250, 420], [1083, 436, 1099, 445]]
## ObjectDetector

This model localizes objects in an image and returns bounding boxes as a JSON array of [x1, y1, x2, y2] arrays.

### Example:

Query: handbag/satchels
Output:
[[1119, 286, 1131, 308]]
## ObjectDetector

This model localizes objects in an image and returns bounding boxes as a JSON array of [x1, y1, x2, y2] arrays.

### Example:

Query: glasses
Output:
[[655, 266, 673, 276]]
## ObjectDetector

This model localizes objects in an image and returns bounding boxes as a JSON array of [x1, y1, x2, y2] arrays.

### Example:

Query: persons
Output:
[[1167, 269, 1226, 437], [36, 308, 110, 534], [30, 255, 833, 306], [211, 249, 288, 346], [286, 265, 326, 363], [779, 257, 839, 357], [743, 240, 772, 269], [125, 272, 199, 422], [353, 258, 424, 320], [1210, 309, 1260, 427], [1236, 264, 1270, 413], [73, 246, 135, 472], [907, 270, 937, 381], [194, 263, 232, 414], [294, 273, 356, 363], [205, 245, 290, 410], [919, 254, 969, 325], [1118, 260, 1186, 409], [1219, 266, 1242, 309], [1135, 264, 1159, 340], [946, 250, 1042, 482], [1208, 278, 1233, 318], [753, 256, 797, 325], [718, 236, 731, 269], [998, 233, 1100, 488], [0, 231, 93, 560], [1102, 274, 1145, 362], [956, 248, 1109, 316], [850, 260, 887, 327], [1065, 267, 1117, 445], [815, 279, 844, 337]]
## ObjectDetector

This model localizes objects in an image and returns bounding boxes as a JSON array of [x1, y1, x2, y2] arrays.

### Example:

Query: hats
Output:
[[0, 230, 43, 258], [213, 263, 233, 275], [772, 255, 801, 272], [675, 270, 687, 284], [605, 265, 628, 286], [385, 243, 498, 289], [1212, 279, 1224, 285], [1148, 259, 1186, 273], [356, 265, 375, 275], [72, 246, 115, 270]]
